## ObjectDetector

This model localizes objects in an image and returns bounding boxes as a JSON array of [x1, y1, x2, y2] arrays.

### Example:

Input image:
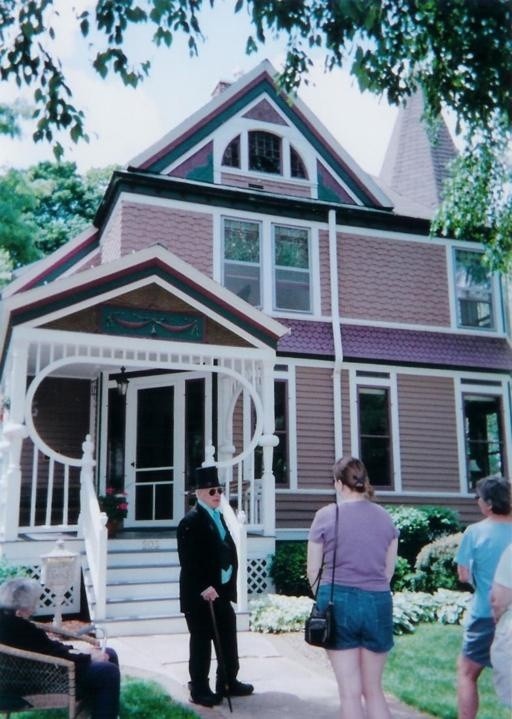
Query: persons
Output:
[[451, 475, 512, 718], [488, 540, 512, 708], [2, 576, 122, 718], [179, 466, 253, 707], [305, 456, 401, 718]]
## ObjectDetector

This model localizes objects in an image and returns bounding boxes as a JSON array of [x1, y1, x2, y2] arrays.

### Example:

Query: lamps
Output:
[[466, 458, 480, 472], [114, 364, 130, 395]]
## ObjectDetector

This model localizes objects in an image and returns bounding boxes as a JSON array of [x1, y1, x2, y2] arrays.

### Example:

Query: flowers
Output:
[[94, 487, 129, 519]]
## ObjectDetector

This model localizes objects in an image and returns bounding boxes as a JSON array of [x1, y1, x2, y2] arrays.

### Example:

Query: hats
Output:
[[192, 466, 221, 489]]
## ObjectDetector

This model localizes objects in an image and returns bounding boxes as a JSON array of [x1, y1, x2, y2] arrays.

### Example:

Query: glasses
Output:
[[209, 489, 222, 496]]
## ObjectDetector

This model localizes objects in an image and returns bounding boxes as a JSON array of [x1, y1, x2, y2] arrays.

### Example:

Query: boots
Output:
[[188, 677, 223, 706], [217, 676, 254, 696]]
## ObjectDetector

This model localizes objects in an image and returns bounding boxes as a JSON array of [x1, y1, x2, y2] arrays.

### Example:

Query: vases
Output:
[[105, 520, 116, 538]]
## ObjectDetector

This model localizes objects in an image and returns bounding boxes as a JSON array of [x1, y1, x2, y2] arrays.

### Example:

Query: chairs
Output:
[[182, 478, 251, 521], [2, 625, 102, 718]]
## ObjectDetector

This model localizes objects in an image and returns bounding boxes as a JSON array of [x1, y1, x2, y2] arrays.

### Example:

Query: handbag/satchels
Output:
[[305, 602, 335, 649]]
